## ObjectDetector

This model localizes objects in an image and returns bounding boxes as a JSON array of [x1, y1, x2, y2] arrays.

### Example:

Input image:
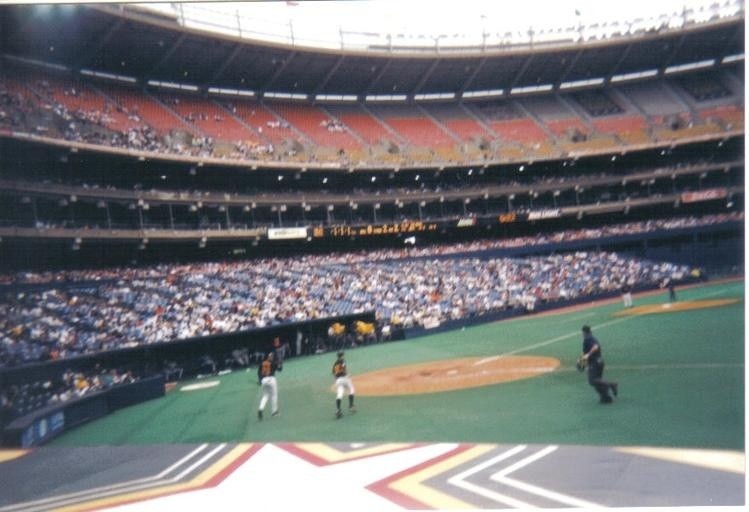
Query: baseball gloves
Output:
[[575, 357, 587, 372]]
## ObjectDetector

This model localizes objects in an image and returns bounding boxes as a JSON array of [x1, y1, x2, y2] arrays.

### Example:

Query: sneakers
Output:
[[348, 406, 356, 412], [610, 381, 618, 397], [599, 397, 612, 404], [258, 410, 263, 419], [271, 410, 279, 416], [335, 408, 343, 418]]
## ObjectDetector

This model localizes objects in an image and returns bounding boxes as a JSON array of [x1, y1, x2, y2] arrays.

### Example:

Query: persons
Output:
[[0, 65, 744, 421]]
